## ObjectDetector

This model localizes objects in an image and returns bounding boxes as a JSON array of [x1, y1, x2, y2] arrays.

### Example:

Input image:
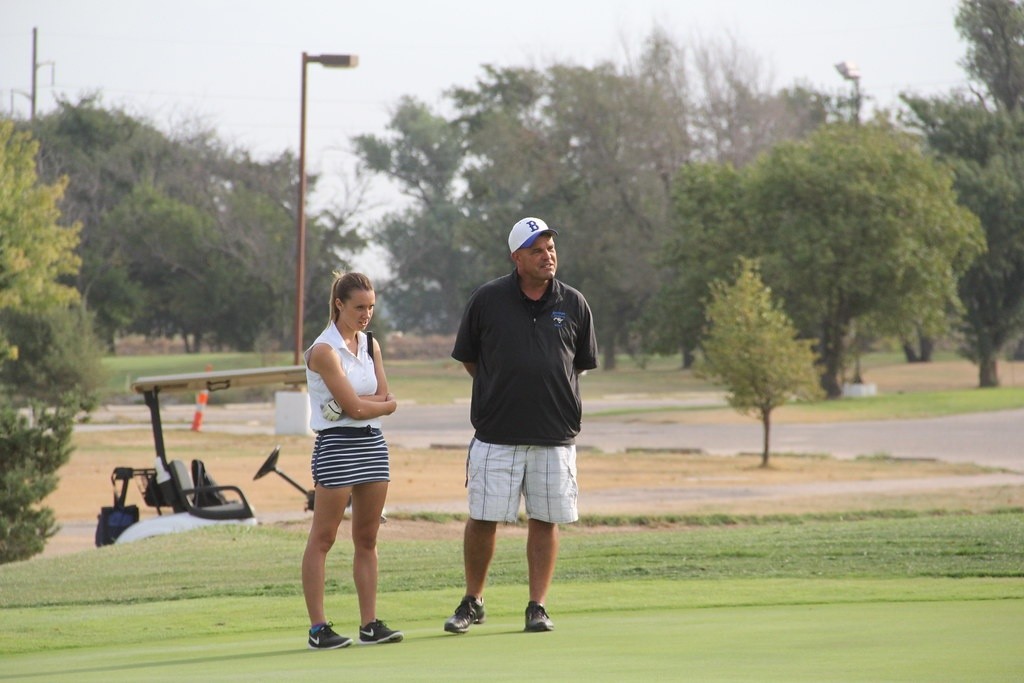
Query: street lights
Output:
[[296, 52, 361, 364]]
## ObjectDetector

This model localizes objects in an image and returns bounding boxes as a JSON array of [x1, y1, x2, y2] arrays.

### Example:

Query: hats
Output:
[[508, 217, 558, 255]]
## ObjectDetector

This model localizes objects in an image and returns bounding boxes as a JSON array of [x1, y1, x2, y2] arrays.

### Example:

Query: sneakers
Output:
[[359, 619, 403, 644], [445, 595, 485, 633], [308, 621, 354, 650], [525, 601, 553, 631]]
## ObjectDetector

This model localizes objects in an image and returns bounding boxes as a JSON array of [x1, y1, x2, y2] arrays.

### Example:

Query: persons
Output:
[[301, 273, 403, 648], [443, 217, 601, 634]]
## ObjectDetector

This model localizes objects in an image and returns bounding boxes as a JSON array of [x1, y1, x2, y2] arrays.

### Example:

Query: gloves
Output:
[[323, 399, 343, 421]]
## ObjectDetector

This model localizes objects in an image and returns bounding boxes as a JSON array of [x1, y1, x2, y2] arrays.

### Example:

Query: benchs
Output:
[[168, 460, 254, 521]]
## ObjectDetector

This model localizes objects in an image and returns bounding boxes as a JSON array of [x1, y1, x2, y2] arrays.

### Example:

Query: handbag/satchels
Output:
[[95, 468, 140, 548]]
